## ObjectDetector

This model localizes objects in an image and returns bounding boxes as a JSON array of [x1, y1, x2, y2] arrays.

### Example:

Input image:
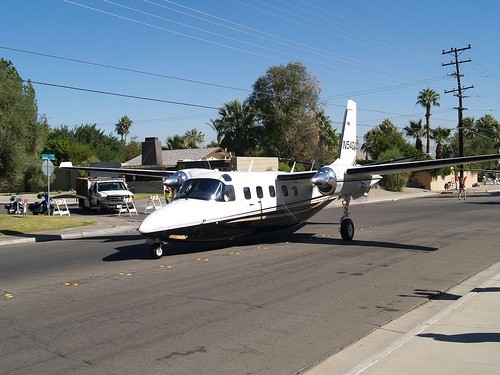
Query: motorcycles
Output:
[[4, 196, 25, 215], [28, 193, 54, 215]]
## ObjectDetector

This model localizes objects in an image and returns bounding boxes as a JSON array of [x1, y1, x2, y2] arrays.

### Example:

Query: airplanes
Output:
[[56, 98, 500, 259]]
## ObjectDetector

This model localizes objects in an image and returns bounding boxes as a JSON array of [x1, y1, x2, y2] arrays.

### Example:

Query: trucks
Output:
[[73, 173, 135, 213]]
